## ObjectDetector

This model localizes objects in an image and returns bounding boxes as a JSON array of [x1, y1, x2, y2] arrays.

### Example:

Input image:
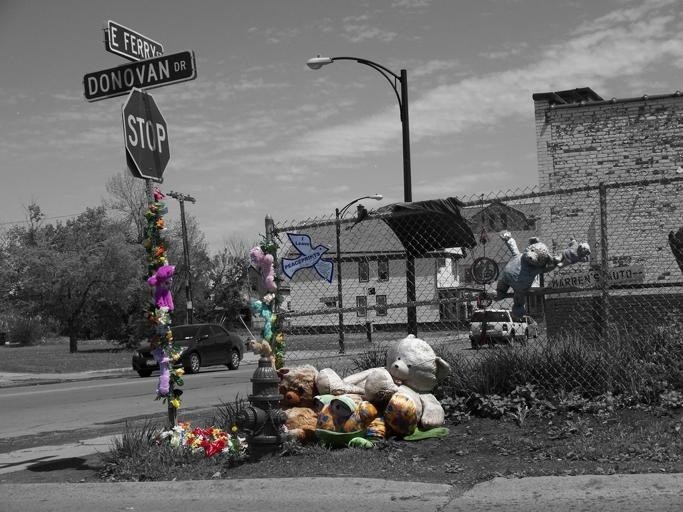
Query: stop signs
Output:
[[118, 84, 171, 182]]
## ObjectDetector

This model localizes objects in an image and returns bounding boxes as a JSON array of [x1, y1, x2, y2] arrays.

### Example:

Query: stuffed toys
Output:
[[495, 230, 555, 317], [246, 296, 277, 341], [151, 345, 172, 399], [314, 332, 452, 428], [248, 245, 278, 292], [553, 235, 591, 269], [146, 265, 175, 312], [313, 392, 449, 449], [281, 403, 319, 443], [274, 363, 318, 406], [249, 339, 275, 368]]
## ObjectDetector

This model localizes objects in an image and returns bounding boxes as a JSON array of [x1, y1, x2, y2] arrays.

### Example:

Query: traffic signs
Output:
[[104, 17, 165, 63], [78, 47, 197, 103]]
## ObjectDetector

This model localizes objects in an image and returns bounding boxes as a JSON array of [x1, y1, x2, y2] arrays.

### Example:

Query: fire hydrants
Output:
[[236, 345, 287, 461], [243, 335, 252, 352]]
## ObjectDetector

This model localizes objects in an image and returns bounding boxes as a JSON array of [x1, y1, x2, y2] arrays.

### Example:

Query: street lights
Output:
[[302, 51, 418, 340], [334, 192, 384, 353]]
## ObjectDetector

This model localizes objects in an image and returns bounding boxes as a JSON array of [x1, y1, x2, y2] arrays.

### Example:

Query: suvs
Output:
[[467, 307, 529, 350]]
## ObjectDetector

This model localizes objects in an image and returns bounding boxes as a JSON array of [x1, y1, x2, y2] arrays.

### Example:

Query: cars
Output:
[[522, 313, 539, 342], [129, 322, 246, 380]]
